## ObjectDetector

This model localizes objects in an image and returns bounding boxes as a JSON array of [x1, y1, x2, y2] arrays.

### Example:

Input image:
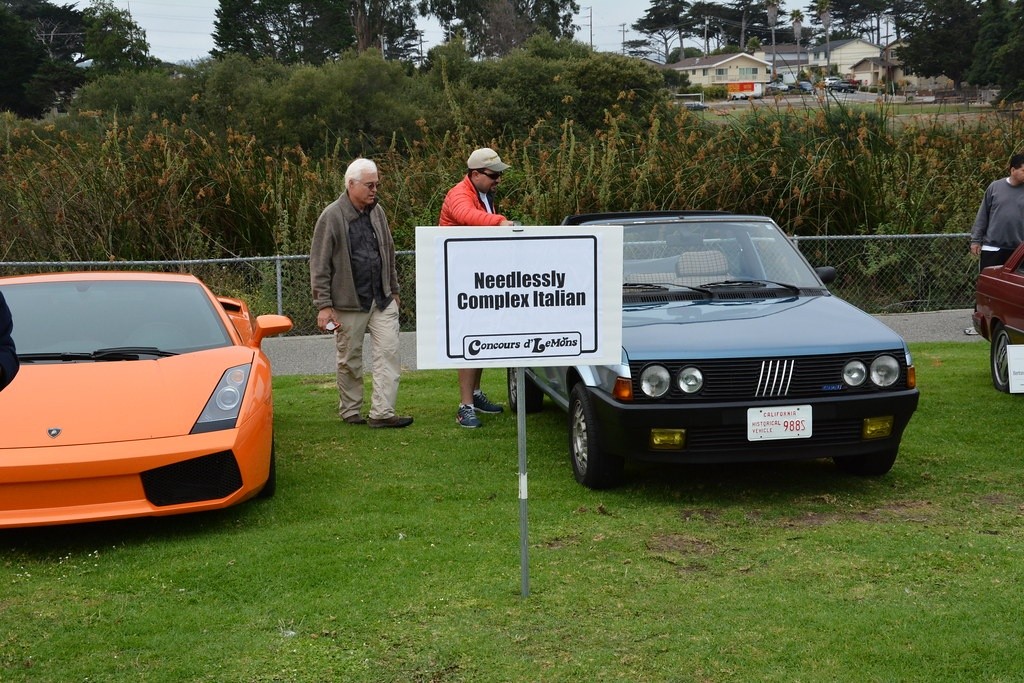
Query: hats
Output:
[[467, 148, 511, 172]]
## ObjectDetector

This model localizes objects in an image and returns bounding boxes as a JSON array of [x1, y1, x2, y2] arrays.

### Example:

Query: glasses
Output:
[[471, 169, 503, 180], [352, 178, 381, 191]]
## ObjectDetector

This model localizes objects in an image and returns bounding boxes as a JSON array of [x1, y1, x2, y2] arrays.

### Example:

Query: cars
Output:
[[789, 81, 817, 94], [970, 238, 1024, 392], [731, 90, 760, 99], [509, 211, 919, 489], [769, 82, 789, 91], [687, 102, 709, 111]]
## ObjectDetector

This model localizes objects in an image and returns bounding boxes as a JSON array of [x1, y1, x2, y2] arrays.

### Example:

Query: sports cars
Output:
[[0, 272, 295, 529]]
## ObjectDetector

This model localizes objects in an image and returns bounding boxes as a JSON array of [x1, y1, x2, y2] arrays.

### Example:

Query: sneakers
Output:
[[473, 391, 503, 414], [456, 404, 482, 428]]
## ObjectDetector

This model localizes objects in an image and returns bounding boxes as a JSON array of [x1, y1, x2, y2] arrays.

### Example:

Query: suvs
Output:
[[821, 76, 839, 86]]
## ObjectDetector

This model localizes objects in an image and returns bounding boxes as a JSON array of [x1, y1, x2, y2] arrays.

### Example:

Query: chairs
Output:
[[672, 251, 736, 290]]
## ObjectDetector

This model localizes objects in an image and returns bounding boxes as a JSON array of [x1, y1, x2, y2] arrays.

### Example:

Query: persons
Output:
[[437, 147, 518, 429], [964, 152, 1024, 336], [309, 157, 415, 430]]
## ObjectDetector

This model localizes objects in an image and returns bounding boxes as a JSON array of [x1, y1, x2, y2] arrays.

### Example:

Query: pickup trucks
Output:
[[828, 80, 857, 93]]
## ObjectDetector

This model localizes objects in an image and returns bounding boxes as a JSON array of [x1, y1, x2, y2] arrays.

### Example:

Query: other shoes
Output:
[[368, 413, 413, 428], [344, 414, 367, 424], [964, 327, 979, 335]]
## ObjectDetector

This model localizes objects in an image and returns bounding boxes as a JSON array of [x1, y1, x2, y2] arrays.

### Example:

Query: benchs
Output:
[[624, 272, 676, 288]]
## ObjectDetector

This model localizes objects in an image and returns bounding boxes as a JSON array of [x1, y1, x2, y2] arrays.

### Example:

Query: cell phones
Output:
[[325, 319, 340, 332]]
